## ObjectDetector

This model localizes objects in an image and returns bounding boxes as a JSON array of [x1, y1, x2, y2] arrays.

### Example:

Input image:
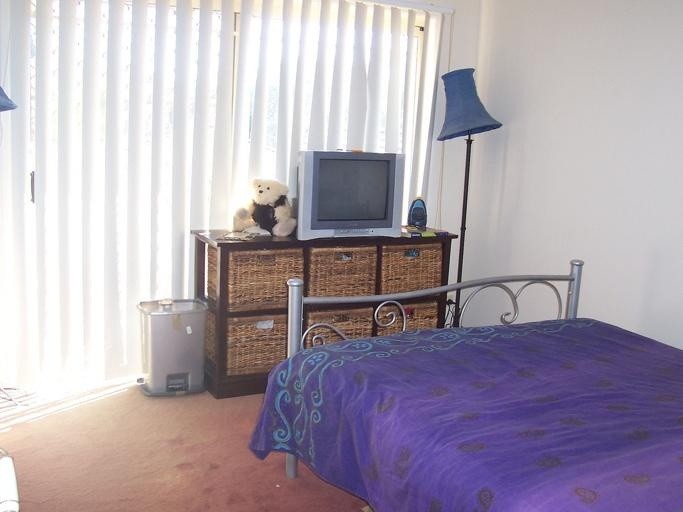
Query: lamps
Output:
[[434, 66, 503, 327]]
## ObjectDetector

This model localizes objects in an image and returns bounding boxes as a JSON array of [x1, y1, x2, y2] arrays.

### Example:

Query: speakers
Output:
[[409, 199, 428, 230]]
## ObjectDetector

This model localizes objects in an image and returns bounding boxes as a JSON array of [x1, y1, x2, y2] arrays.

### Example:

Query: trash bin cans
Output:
[[136, 299, 210, 397]]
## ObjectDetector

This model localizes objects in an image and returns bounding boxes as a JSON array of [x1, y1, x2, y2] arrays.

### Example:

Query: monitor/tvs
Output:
[[296, 149, 406, 242]]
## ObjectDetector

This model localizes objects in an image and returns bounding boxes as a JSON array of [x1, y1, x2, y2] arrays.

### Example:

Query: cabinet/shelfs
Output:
[[188, 227, 460, 402]]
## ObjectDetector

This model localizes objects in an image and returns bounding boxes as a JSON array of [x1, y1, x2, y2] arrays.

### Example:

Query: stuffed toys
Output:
[[233, 178, 296, 237]]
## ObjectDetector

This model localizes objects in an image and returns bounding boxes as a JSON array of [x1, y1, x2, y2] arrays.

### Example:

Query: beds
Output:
[[272, 259, 682, 512]]
